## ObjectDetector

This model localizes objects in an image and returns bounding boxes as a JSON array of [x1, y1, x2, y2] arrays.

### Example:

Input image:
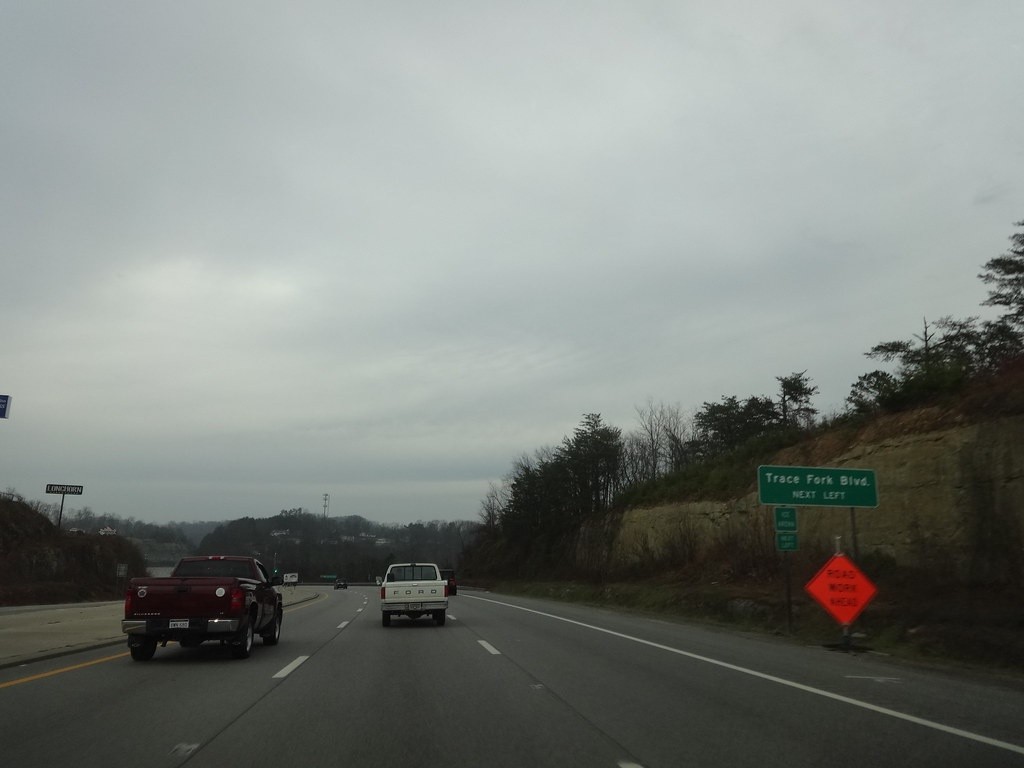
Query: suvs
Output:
[[334, 578, 348, 589], [439, 571, 457, 595]]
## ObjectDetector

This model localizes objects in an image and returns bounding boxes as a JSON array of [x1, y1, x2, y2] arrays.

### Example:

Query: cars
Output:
[[377, 578, 382, 585]]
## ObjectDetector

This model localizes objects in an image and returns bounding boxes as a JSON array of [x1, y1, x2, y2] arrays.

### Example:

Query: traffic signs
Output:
[[756, 466, 879, 508]]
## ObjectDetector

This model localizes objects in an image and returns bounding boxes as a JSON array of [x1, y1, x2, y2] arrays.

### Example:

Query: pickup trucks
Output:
[[379, 562, 449, 625], [122, 556, 282, 654]]
